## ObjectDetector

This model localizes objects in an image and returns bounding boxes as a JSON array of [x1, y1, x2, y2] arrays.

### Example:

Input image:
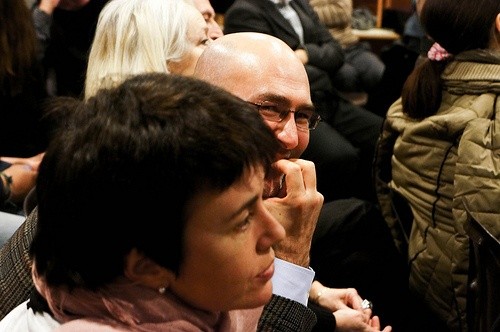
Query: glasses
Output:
[[246, 101, 321, 131]]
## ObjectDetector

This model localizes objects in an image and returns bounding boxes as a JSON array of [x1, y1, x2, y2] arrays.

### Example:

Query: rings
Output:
[[362, 298, 373, 309]]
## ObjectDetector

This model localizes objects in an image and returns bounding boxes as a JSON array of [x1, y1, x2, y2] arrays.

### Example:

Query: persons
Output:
[[0, 30, 323, 332], [376, 0, 500, 332], [0, 0, 437, 252], [0, 73, 287, 332]]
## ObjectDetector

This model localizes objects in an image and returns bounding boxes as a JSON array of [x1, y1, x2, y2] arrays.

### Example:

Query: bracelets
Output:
[[344, 288, 354, 305], [314, 285, 326, 304]]
